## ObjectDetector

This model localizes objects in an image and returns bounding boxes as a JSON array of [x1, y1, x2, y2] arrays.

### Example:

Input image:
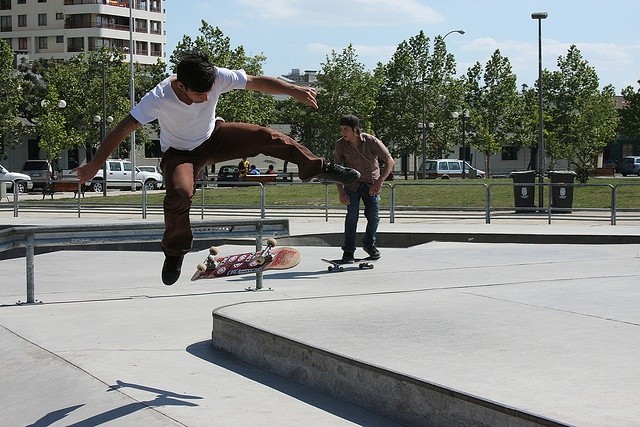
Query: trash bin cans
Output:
[[510, 170, 537, 212], [548, 170, 578, 213]]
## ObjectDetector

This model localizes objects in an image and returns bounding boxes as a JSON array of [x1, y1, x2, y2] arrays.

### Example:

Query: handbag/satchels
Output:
[[372, 168, 380, 184]]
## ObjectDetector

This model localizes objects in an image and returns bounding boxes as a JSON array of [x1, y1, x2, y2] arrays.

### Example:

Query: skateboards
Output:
[[190, 237, 301, 281], [321, 256, 381, 272]]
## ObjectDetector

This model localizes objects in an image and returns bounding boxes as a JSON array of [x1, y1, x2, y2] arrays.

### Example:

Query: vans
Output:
[[417, 159, 486, 180], [619, 156, 640, 176], [21, 160, 52, 192]]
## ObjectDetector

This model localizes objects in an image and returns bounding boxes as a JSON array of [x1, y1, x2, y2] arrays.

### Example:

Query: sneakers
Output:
[[317, 158, 361, 185], [363, 244, 381, 259], [162, 255, 184, 285], [342, 251, 355, 264]]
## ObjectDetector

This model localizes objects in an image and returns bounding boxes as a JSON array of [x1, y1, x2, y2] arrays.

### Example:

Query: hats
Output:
[[340, 115, 359, 128]]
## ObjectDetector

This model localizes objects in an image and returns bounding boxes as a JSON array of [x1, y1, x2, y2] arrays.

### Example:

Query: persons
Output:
[[250, 164, 260, 176], [264, 165, 276, 174], [70, 52, 360, 285], [238, 157, 249, 176], [336, 115, 395, 261]]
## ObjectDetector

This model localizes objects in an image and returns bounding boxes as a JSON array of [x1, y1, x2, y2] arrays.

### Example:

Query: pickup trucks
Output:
[[58, 158, 162, 192]]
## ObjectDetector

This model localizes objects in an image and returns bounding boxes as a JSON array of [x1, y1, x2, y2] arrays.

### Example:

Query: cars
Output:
[[138, 166, 163, 190], [0, 165, 34, 193], [217, 165, 240, 187]]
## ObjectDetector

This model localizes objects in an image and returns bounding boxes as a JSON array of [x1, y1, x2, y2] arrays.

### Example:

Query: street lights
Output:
[[93, 115, 113, 144], [453, 109, 470, 180], [417, 122, 434, 180], [433, 25, 466, 51], [41, 98, 66, 110]]
